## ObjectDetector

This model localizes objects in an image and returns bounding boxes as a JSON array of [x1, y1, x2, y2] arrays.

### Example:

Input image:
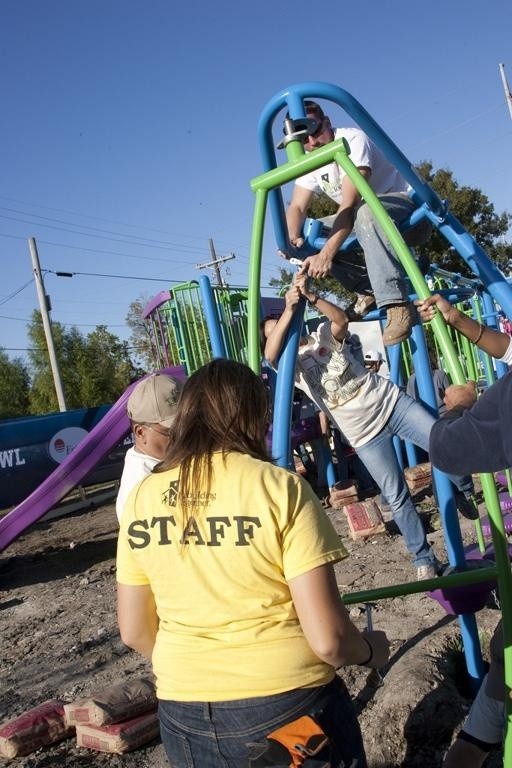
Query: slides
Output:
[[265, 417, 317, 455], [0, 366, 185, 553]]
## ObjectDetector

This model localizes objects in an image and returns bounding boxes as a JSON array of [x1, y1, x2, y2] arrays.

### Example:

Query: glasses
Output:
[[304, 119, 323, 144]]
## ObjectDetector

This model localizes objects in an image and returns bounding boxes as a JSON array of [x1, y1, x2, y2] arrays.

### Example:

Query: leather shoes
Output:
[[345, 290, 376, 321], [381, 304, 417, 346]]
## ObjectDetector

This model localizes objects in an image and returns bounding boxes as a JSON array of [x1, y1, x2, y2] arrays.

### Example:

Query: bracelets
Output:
[[473, 324, 484, 345], [359, 637, 374, 666], [309, 294, 320, 306]]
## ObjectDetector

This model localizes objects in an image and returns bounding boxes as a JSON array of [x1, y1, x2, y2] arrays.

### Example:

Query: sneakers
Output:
[[417, 560, 444, 581], [455, 490, 479, 520]]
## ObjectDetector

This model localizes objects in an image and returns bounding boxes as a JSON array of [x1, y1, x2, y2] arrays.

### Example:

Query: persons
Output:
[[412, 293, 512, 366], [497, 311, 512, 337], [258, 272, 479, 585], [429, 369, 512, 768], [115, 358, 390, 767], [299, 388, 336, 497], [342, 351, 384, 500], [404, 347, 454, 417], [115, 373, 185, 526], [277, 100, 433, 346]]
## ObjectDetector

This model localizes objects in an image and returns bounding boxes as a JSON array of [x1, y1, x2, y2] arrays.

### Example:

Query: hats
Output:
[[127, 373, 179, 428], [364, 350, 386, 362]]
